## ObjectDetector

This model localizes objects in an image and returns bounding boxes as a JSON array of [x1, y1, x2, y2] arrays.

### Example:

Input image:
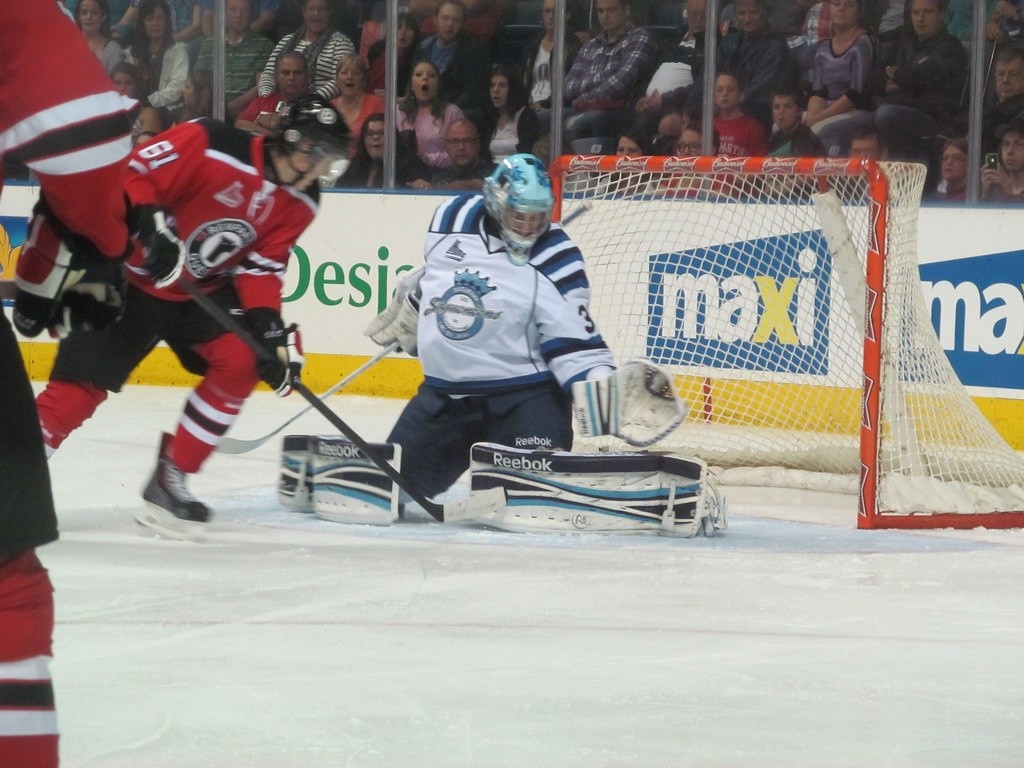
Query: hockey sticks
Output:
[[133, 232, 506, 525], [211, 198, 595, 455]]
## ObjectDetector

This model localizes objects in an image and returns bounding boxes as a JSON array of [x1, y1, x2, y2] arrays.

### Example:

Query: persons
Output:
[[0, 0, 133, 768], [74, 0, 1023, 203], [366, 156, 624, 504], [32, 94, 354, 535]]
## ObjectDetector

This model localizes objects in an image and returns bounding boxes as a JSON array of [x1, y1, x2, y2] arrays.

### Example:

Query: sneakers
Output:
[[135, 432, 210, 542]]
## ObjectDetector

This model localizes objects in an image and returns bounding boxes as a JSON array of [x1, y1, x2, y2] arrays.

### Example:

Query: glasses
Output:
[[939, 154, 967, 163], [446, 137, 479, 148], [674, 141, 702, 153], [363, 129, 384, 138]]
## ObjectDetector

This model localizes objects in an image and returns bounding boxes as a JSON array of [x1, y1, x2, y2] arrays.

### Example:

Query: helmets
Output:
[[482, 154, 555, 264], [275, 93, 354, 183]]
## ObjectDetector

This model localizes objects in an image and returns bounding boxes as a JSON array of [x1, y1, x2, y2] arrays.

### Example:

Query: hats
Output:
[[994, 118, 1024, 143]]
